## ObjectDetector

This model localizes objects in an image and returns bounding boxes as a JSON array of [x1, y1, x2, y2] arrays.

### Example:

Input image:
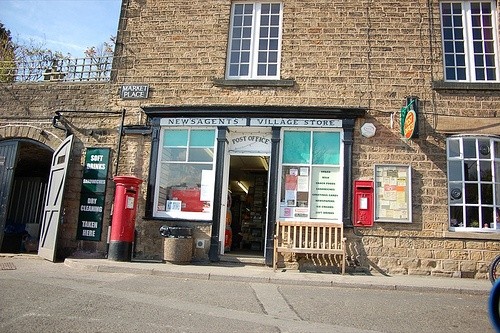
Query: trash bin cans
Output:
[[161, 226, 193, 265]]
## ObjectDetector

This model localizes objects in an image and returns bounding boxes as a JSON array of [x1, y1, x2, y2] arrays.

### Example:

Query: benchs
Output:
[[273, 221, 347, 276]]
